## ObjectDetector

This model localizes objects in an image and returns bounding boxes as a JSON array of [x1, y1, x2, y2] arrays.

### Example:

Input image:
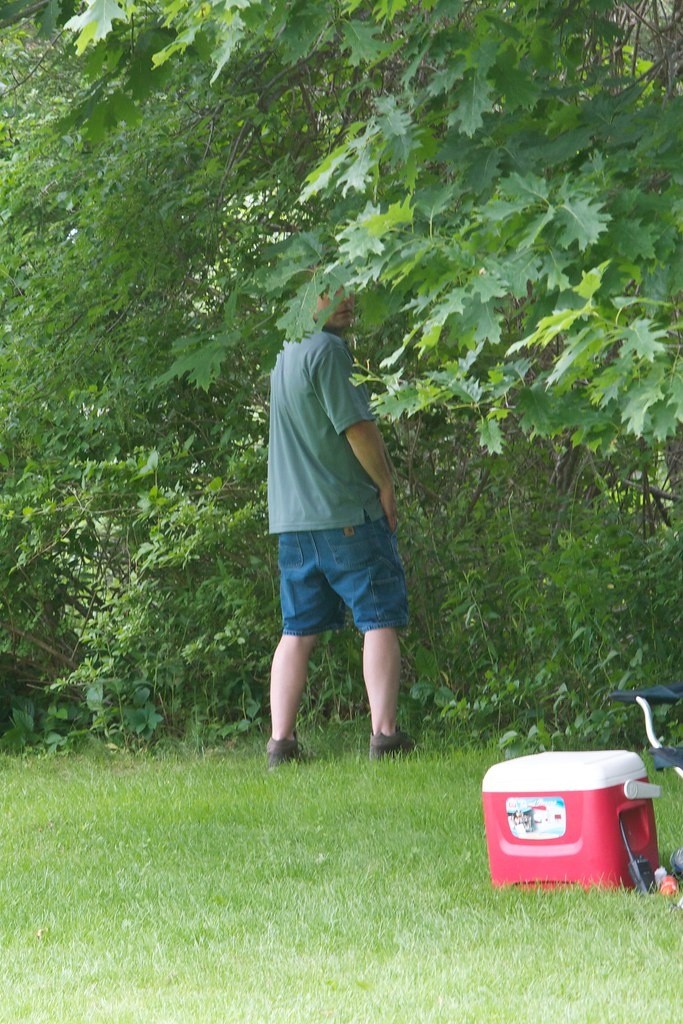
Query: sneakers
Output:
[[369, 725, 416, 751], [267, 737, 303, 756]]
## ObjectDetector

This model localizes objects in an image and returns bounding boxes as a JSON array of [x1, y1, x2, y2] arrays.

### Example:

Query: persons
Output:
[[264, 256, 413, 765]]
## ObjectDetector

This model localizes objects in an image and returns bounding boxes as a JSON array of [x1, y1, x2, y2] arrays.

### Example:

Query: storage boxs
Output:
[[482, 747, 663, 894]]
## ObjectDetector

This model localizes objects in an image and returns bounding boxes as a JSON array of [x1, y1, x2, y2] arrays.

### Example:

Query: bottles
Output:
[[653, 867, 679, 897], [669, 848, 683, 872]]
